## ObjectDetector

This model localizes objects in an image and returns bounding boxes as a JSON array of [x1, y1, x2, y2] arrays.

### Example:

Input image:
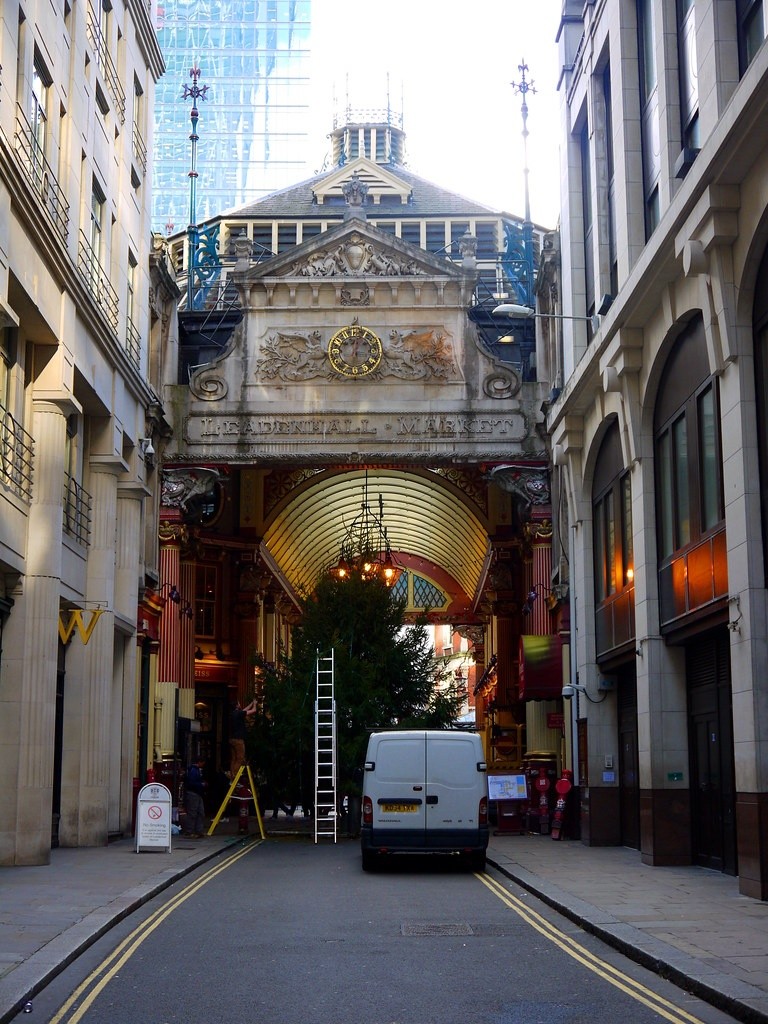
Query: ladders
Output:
[[314, 646, 338, 845], [208, 759, 267, 843]]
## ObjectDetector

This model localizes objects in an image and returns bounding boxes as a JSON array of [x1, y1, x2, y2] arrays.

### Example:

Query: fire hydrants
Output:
[[236, 788, 252, 834]]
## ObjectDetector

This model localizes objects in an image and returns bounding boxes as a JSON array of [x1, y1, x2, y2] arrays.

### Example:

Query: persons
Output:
[[228, 696, 259, 789], [182, 755, 208, 841]]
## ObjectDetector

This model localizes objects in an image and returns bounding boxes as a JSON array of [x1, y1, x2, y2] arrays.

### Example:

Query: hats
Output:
[[195, 756, 206, 761]]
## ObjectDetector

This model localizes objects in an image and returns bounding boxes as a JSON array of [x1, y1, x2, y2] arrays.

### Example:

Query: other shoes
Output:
[[183, 832, 198, 838], [196, 832, 207, 838]]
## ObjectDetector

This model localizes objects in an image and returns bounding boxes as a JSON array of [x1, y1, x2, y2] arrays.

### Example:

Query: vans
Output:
[[359, 730, 489, 874]]
